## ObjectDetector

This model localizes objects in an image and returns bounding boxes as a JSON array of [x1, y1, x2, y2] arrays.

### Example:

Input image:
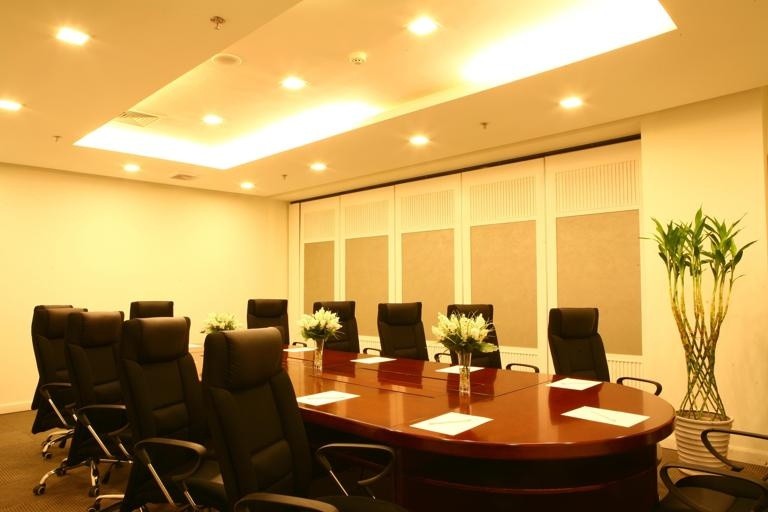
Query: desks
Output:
[[189, 344, 677, 496]]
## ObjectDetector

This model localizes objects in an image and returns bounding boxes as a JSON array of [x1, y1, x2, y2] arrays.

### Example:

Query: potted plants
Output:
[[637, 204, 759, 475]]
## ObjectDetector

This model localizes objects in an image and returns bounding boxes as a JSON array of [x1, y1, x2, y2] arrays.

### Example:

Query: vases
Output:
[[455, 350, 472, 403], [314, 337, 324, 375]]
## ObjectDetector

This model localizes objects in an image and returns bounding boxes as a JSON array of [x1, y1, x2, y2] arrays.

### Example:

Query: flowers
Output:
[[200, 312, 241, 335], [430, 310, 499, 354], [297, 307, 345, 340]]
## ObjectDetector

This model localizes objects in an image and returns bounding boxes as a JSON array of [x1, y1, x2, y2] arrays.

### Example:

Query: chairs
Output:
[[378, 302, 451, 363], [548, 308, 662, 396], [87, 317, 227, 512], [247, 299, 307, 347], [659, 428, 768, 511], [130, 301, 173, 319], [447, 304, 540, 373], [203, 326, 409, 511], [313, 301, 382, 355], [33, 311, 126, 496], [32, 305, 88, 459]]
